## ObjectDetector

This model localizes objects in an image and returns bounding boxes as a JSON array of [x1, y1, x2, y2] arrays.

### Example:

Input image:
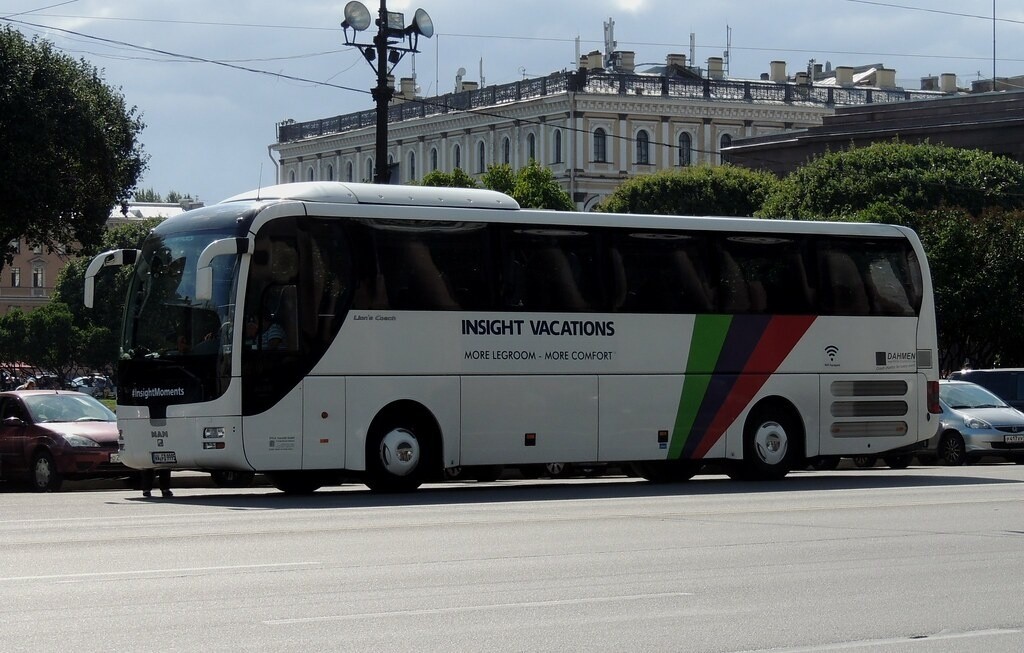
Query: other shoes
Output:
[[143, 489, 150, 496], [162, 490, 173, 497]]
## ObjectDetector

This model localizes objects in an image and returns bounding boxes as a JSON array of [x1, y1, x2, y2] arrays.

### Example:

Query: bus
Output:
[[82, 180, 939, 495], [947, 367, 1024, 414]]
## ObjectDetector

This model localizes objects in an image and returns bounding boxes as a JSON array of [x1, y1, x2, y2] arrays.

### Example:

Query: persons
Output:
[[15, 378, 37, 390], [93, 387, 110, 399], [244, 309, 288, 351], [143, 469, 173, 498]]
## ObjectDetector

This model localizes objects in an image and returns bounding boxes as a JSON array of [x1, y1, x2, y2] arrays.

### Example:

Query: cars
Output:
[[0, 370, 115, 398], [0, 391, 152, 494], [853, 378, 1024, 465]]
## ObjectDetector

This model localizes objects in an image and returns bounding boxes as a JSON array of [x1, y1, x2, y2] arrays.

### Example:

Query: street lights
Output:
[[340, 0, 434, 183]]
